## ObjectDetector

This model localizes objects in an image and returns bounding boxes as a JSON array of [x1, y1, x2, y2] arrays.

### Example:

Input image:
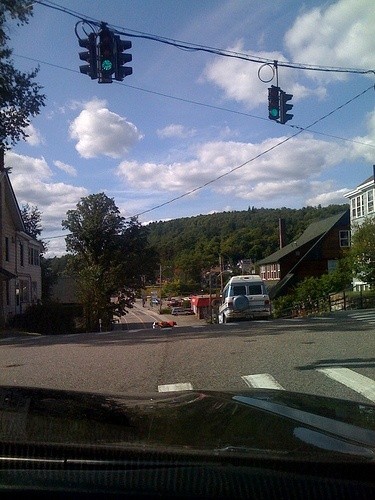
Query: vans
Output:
[[219, 275, 271, 323]]
